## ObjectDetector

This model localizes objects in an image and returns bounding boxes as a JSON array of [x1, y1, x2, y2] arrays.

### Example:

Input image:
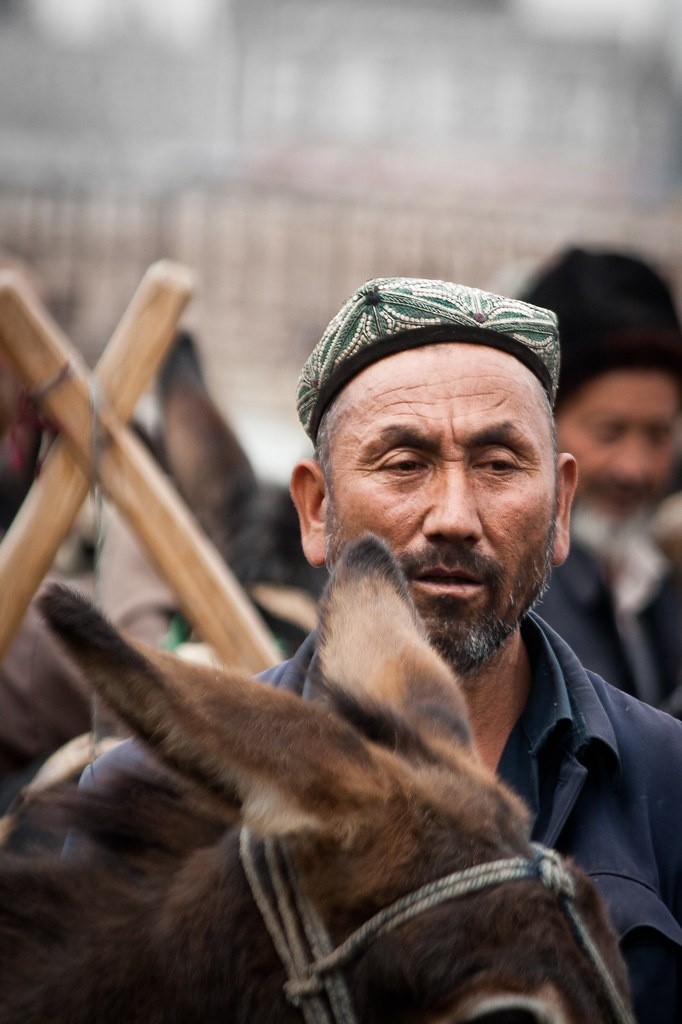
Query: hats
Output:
[[296, 277, 561, 448], [513, 247, 682, 410]]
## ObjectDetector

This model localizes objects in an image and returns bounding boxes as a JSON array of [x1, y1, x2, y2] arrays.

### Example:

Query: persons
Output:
[[514, 246, 681, 722], [59, 278, 682, 1024]]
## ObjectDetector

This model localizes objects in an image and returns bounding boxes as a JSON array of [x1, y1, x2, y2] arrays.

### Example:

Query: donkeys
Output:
[[0, 326, 637, 1024]]
